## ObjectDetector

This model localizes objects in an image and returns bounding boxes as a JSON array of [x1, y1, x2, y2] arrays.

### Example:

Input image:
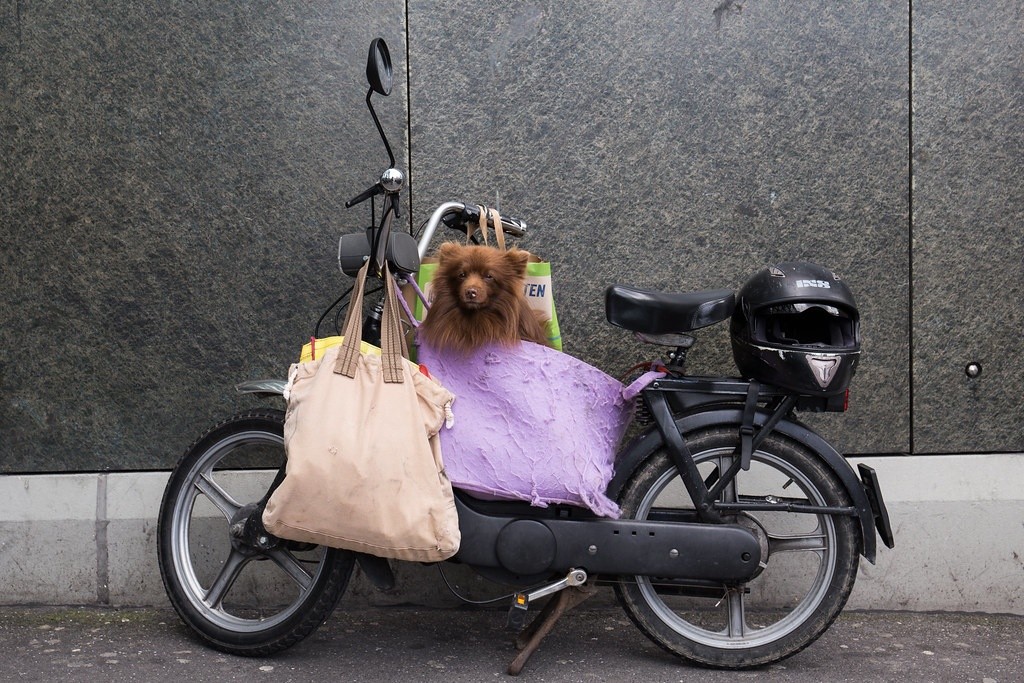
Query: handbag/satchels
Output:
[[262, 253, 462, 563], [413, 204, 562, 353]]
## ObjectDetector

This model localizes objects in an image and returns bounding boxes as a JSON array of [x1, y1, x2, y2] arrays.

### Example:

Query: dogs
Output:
[[417, 240, 556, 360]]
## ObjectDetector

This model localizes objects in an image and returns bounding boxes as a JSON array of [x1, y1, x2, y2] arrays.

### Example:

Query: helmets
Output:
[[730, 262, 862, 398]]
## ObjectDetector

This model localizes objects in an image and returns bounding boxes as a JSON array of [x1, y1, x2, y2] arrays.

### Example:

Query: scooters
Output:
[[152, 36, 898, 683]]
